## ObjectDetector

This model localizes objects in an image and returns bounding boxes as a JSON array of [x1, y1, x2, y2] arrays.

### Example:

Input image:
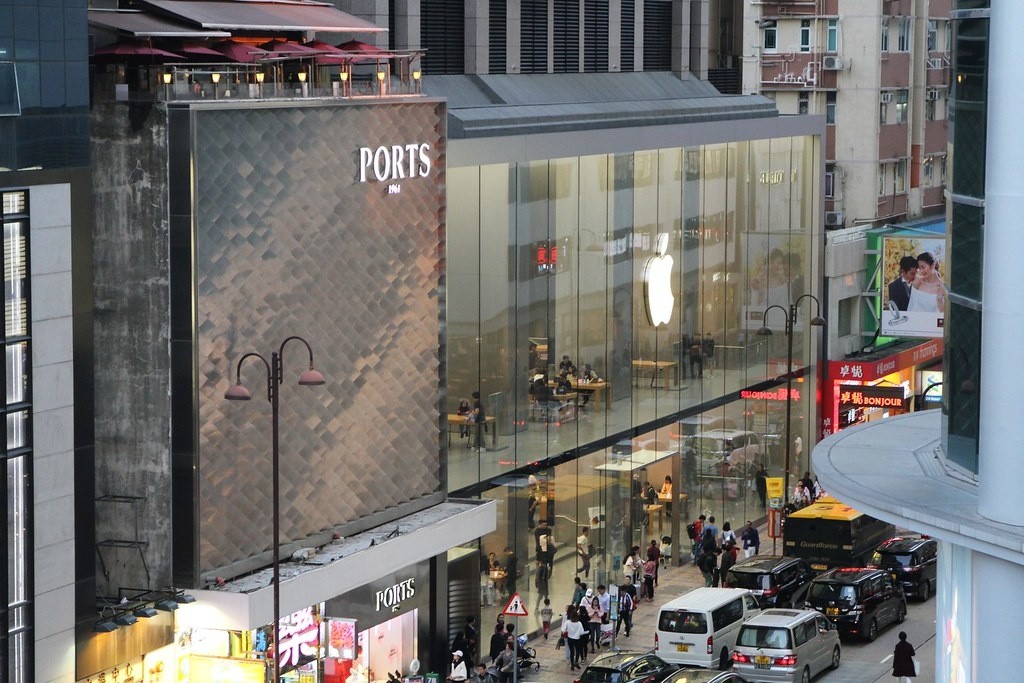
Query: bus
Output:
[[783, 496, 895, 576]]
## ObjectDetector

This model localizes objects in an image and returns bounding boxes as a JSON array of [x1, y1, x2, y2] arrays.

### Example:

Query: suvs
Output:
[[867, 538, 937, 603], [661, 667, 748, 683]]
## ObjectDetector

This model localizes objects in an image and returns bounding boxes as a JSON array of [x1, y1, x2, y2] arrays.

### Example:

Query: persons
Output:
[[756, 465, 769, 508], [446, 613, 521, 683], [534, 519, 670, 671], [892, 631, 917, 683], [458, 392, 488, 453], [534, 355, 597, 414], [632, 474, 673, 531], [784, 472, 825, 518], [888, 252, 948, 312], [755, 249, 805, 313], [688, 332, 715, 379], [691, 515, 760, 589], [480, 547, 517, 607], [529, 493, 538, 531]]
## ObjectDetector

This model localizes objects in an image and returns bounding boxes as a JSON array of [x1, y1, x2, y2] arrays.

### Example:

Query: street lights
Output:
[[758, 294, 827, 506], [224, 336, 325, 683]]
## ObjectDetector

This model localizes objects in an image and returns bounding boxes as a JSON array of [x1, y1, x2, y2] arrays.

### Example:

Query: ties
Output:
[[622, 596, 624, 611]]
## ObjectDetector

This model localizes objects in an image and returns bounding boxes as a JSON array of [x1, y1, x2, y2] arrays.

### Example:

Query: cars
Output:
[[493, 402, 595, 467], [670, 416, 738, 448], [574, 653, 680, 683], [748, 412, 803, 465], [608, 438, 666, 462]]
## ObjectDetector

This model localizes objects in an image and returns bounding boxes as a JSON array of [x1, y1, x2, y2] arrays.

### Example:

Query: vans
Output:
[[654, 586, 761, 670], [723, 554, 816, 611], [804, 567, 907, 642], [733, 607, 841, 683], [684, 429, 759, 479]]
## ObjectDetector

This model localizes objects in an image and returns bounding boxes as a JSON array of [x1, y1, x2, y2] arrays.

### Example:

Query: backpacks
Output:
[[687, 521, 700, 539], [695, 553, 706, 567]]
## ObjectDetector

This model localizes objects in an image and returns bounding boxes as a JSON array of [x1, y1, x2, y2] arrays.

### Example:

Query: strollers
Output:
[[486, 666, 502, 683], [513, 647, 541, 673]]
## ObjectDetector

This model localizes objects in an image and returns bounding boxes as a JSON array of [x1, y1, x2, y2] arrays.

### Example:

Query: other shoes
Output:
[[575, 663, 581, 669], [581, 661, 585, 664], [616, 632, 618, 637], [625, 632, 630, 637], [571, 664, 575, 670], [585, 657, 587, 662], [595, 642, 601, 649], [591, 647, 595, 652], [545, 633, 548, 639]]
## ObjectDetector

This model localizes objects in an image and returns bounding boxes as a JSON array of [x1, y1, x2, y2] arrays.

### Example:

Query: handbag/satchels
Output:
[[546, 544, 557, 560], [912, 656, 920, 676], [555, 631, 568, 650], [730, 530, 736, 545], [588, 544, 596, 559]]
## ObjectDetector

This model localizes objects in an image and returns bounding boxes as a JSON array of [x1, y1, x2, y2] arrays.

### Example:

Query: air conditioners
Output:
[[882, 93, 894, 102], [823, 56, 843, 69], [825, 212, 842, 225], [930, 58, 942, 68], [928, 91, 939, 100]]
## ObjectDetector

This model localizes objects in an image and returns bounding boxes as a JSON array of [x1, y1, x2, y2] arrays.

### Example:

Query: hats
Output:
[[585, 588, 593, 597], [452, 650, 463, 657]]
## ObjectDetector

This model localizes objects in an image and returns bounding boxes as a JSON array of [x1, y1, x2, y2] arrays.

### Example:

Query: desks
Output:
[[447, 413, 496, 447], [550, 379, 612, 422], [656, 493, 689, 518], [643, 504, 663, 535], [489, 570, 508, 599], [632, 360, 679, 390]]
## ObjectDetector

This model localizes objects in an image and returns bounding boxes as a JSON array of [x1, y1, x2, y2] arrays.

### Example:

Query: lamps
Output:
[[212, 73, 220, 83], [164, 72, 171, 84], [92, 591, 197, 633], [340, 72, 348, 81], [256, 72, 265, 83], [298, 72, 307, 83], [378, 71, 386, 81], [413, 71, 420, 80]]
